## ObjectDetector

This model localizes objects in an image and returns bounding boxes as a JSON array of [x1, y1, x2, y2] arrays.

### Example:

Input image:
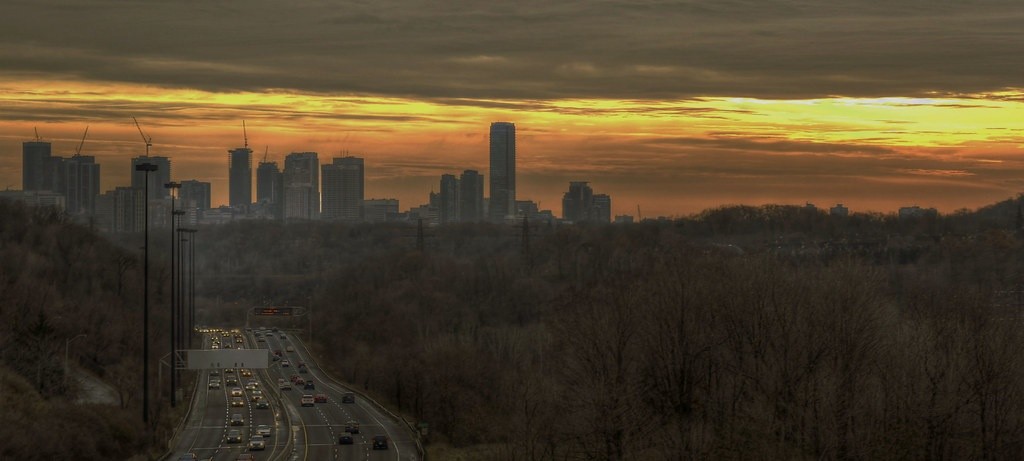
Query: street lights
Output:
[[136, 160, 199, 427]]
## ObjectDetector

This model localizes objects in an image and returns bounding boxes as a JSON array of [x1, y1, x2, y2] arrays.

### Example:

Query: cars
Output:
[[190, 320, 395, 460]]
